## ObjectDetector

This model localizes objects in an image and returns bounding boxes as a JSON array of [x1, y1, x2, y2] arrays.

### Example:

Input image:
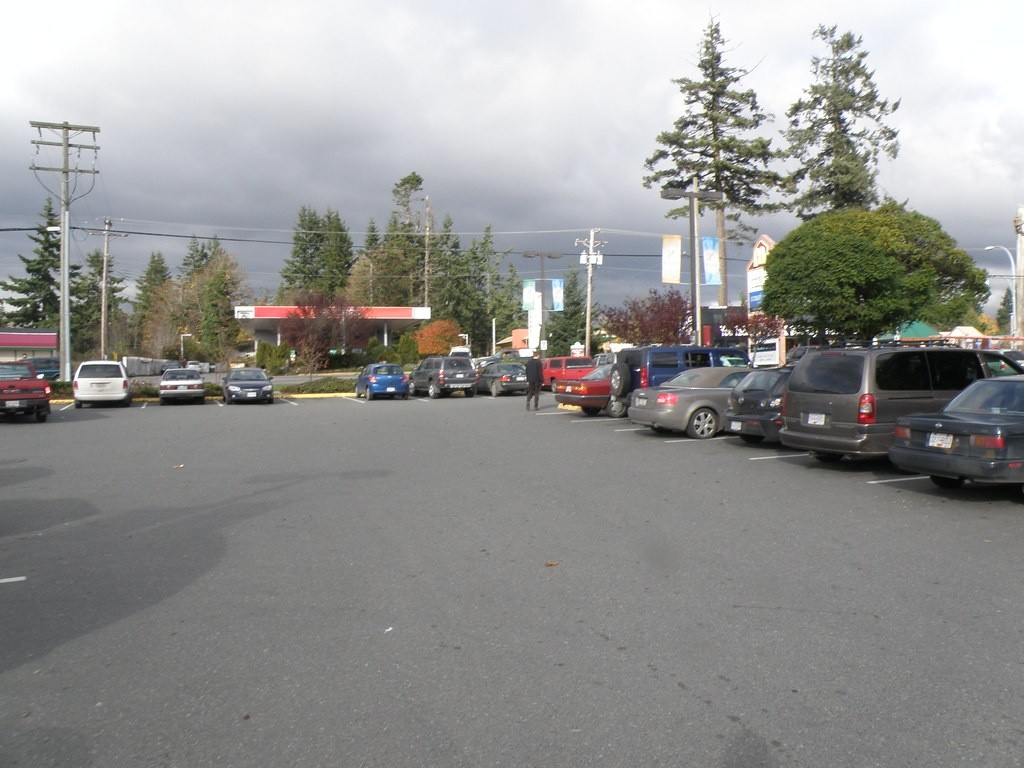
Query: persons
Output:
[[20, 353, 27, 360], [525, 352, 544, 411]]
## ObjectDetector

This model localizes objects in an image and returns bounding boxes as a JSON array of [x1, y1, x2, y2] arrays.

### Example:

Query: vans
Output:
[[778, 338, 1024, 475], [448, 347, 472, 359], [477, 350, 535, 369]]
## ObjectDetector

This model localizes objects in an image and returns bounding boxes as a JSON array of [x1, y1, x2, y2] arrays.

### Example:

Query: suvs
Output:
[[610, 345, 753, 412], [407, 355, 478, 399]]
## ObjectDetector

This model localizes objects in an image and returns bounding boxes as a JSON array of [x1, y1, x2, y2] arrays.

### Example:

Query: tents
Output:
[[878, 319, 984, 341]]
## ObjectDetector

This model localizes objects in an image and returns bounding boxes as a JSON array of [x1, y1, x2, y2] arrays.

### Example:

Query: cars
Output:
[[354, 363, 410, 400], [222, 367, 274, 404], [786, 344, 819, 365], [0, 360, 52, 423], [986, 349, 1024, 377], [472, 363, 531, 397], [185, 361, 202, 374], [159, 360, 180, 374], [626, 367, 757, 439], [19, 356, 75, 383], [723, 361, 797, 449], [72, 359, 135, 408], [886, 376, 1024, 499], [540, 353, 629, 419], [159, 369, 205, 405]]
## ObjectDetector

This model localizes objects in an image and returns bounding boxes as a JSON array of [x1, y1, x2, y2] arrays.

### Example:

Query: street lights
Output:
[[983, 246, 1016, 337], [661, 187, 724, 343], [180, 333, 193, 368], [522, 249, 563, 359]]
